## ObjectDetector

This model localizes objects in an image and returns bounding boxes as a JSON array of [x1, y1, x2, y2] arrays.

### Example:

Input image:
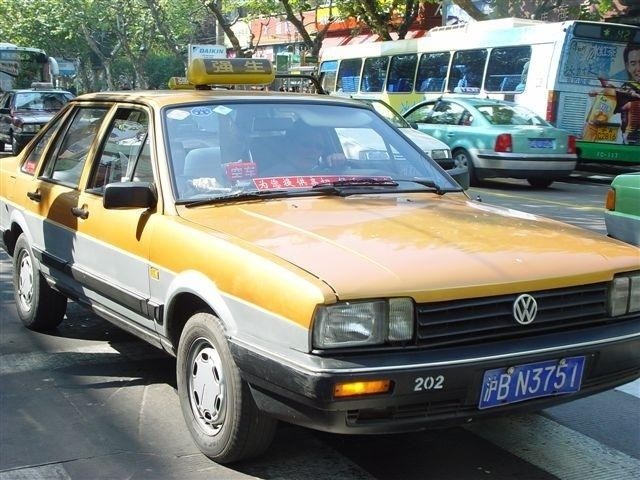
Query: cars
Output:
[[394, 97, 580, 190], [328, 95, 472, 195], [602, 172, 640, 254], [0, 56, 640, 463], [1, 81, 79, 156]]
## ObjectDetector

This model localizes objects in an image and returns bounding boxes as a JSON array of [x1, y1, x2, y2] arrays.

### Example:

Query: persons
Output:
[[613, 42, 640, 144], [621, 91, 640, 144], [257, 119, 346, 178]]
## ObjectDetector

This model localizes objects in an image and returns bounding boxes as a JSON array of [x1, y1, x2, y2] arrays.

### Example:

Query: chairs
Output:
[[183, 146, 219, 178], [241, 134, 284, 177]]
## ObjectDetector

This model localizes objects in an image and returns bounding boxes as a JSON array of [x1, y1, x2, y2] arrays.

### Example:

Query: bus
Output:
[[315, 15, 640, 174], [1, 43, 60, 96]]
